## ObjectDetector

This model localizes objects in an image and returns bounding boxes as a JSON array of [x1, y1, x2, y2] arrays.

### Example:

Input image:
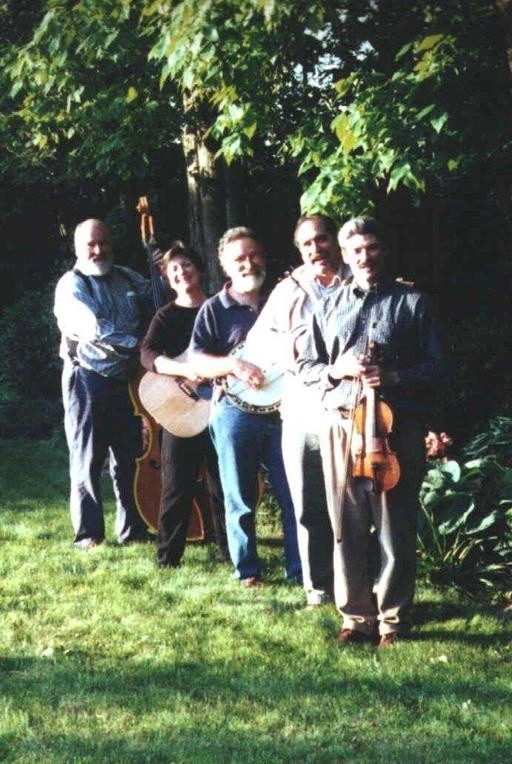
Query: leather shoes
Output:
[[339, 630, 354, 640], [242, 577, 256, 589], [379, 635, 401, 649]]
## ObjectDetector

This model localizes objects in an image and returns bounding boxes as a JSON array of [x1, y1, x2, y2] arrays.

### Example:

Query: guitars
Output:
[[138, 347, 213, 439]]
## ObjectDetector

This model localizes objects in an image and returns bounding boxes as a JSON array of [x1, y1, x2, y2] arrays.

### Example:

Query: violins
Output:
[[350, 341, 403, 494]]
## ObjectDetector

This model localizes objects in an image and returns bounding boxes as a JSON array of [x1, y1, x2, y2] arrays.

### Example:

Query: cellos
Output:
[[127, 195, 265, 541]]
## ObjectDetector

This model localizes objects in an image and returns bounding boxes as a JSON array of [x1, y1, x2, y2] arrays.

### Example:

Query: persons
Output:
[[53, 218, 164, 546], [295, 216, 444, 643], [187, 226, 304, 585], [142, 242, 231, 567], [248, 212, 354, 606]]
